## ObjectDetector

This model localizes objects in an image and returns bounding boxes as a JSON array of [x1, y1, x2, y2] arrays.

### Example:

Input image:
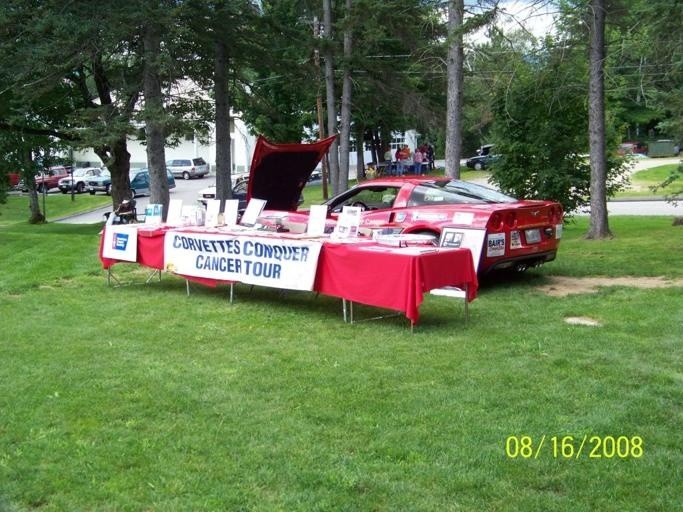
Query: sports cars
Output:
[[243, 174, 567, 277]]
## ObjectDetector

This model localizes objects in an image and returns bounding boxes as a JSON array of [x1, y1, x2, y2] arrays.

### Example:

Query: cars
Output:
[[615, 140, 649, 157], [196, 172, 305, 215], [16, 165, 176, 200], [166, 157, 210, 180], [466, 151, 498, 170]]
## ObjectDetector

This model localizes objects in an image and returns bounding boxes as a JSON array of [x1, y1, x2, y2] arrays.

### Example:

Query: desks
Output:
[[100, 219, 472, 335]]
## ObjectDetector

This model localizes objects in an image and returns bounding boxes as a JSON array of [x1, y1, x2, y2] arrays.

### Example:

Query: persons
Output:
[[97, 198, 135, 237], [383, 141, 436, 176]]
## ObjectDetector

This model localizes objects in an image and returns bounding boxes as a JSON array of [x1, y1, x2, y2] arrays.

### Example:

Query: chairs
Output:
[[145, 196, 361, 303]]
